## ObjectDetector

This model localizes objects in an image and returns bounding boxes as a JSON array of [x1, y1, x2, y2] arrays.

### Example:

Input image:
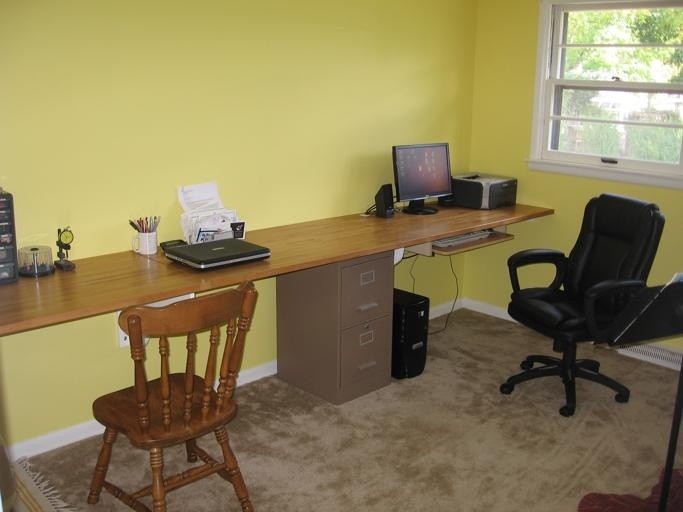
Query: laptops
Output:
[[164, 238, 272, 269]]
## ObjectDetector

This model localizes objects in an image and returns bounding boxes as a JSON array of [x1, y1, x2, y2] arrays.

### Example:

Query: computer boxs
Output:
[[392, 287, 430, 379]]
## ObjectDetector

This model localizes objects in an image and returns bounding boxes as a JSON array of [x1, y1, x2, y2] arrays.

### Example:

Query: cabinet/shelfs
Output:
[[275, 248, 394, 406]]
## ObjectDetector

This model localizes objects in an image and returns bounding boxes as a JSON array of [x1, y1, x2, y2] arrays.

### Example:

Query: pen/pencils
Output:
[[129, 216, 161, 232]]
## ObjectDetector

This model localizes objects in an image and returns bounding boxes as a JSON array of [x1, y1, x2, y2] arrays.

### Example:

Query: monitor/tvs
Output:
[[391, 142, 454, 214]]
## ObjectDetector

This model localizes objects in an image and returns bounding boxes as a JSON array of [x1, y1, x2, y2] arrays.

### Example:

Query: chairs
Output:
[[86, 281, 257, 512], [499, 192, 665, 417]]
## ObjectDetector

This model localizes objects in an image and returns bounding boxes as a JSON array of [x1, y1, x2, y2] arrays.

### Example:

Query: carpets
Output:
[[1, 455, 78, 512]]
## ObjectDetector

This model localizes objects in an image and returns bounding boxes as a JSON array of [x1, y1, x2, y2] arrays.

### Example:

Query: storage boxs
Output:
[[0, 192, 19, 285]]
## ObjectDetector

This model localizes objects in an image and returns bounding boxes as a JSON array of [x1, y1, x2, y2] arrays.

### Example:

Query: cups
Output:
[[131, 231, 158, 255]]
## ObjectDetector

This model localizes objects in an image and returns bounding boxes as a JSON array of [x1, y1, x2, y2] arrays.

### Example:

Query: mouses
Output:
[[488, 228, 496, 234]]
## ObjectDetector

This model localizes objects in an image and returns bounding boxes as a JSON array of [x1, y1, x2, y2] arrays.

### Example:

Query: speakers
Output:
[[375, 183, 394, 218]]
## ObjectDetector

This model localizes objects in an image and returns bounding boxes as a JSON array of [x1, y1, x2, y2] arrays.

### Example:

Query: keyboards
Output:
[[432, 230, 489, 248]]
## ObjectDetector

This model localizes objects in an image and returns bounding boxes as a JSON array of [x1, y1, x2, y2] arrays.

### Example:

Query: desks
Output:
[[0, 196, 554, 337]]
